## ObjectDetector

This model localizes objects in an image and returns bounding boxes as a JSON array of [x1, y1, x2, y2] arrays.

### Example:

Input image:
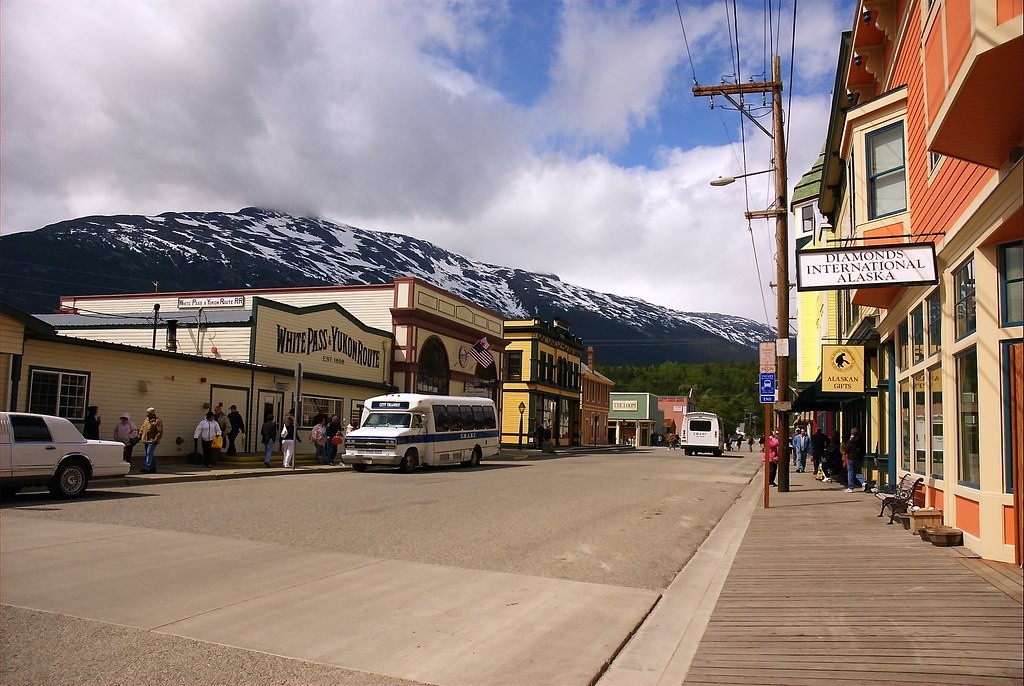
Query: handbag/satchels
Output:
[[212, 435, 223, 449], [281, 424, 288, 438], [130, 430, 138, 446], [332, 435, 342, 445]]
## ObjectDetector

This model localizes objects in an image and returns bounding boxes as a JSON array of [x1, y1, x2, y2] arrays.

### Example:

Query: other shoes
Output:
[[845, 488, 853, 492], [264, 462, 272, 467], [862, 483, 867, 491]]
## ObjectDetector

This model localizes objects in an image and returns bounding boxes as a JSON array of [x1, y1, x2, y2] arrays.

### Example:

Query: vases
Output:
[[898, 511, 910, 530], [918, 526, 963, 547], [907, 507, 943, 534]]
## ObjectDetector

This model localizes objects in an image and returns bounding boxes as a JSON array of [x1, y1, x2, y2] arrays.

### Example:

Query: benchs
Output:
[[875, 474, 924, 525]]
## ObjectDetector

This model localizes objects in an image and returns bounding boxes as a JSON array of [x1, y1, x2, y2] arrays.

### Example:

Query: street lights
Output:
[[710, 168, 775, 186], [518, 402, 525, 449]]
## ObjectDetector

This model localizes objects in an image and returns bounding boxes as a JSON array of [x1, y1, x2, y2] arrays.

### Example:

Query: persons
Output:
[[669, 433, 680, 451], [788, 427, 850, 483], [113, 412, 141, 471], [726, 435, 742, 452], [261, 414, 276, 467], [660, 433, 666, 447], [279, 408, 301, 467], [748, 434, 754, 452], [538, 424, 551, 449], [194, 405, 246, 470], [139, 407, 163, 473], [759, 429, 779, 486], [312, 407, 342, 466], [842, 427, 868, 492], [82, 406, 101, 440]]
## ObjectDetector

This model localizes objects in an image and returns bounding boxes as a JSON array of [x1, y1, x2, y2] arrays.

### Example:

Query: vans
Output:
[[679, 411, 725, 457], [342, 393, 500, 472]]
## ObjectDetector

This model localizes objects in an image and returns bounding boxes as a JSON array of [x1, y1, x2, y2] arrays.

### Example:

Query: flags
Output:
[[480, 337, 495, 351], [468, 340, 494, 368]]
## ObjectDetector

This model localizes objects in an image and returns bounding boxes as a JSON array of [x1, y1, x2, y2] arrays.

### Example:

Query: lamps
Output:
[[139, 380, 154, 392]]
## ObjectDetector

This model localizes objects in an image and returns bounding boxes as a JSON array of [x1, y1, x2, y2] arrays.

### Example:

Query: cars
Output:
[[0, 411, 131, 499]]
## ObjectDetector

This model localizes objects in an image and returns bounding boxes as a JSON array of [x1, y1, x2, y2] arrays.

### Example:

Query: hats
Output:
[[266, 414, 274, 421], [206, 412, 215, 420], [230, 405, 236, 408], [146, 408, 155, 417]]
